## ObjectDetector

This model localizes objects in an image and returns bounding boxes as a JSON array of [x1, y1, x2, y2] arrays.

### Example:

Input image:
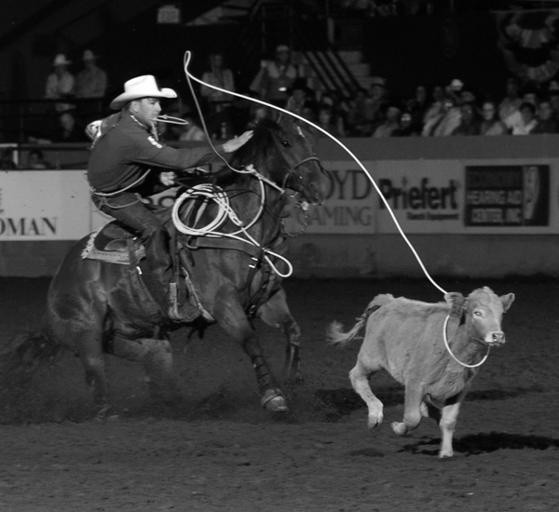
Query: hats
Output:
[[54, 54, 72, 66], [83, 50, 94, 60], [110, 75, 177, 110]]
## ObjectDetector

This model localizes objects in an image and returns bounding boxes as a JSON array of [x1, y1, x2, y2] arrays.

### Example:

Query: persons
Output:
[[85, 74, 255, 329], [44, 51, 107, 140], [164, 44, 559, 145]]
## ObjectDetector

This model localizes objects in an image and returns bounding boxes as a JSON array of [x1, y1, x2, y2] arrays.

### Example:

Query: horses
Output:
[[0, 92, 332, 423]]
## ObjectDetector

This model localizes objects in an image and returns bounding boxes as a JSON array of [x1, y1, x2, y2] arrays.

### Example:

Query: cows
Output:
[[325, 285, 517, 460]]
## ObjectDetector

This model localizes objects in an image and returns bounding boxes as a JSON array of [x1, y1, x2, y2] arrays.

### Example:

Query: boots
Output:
[[142, 231, 201, 321]]
